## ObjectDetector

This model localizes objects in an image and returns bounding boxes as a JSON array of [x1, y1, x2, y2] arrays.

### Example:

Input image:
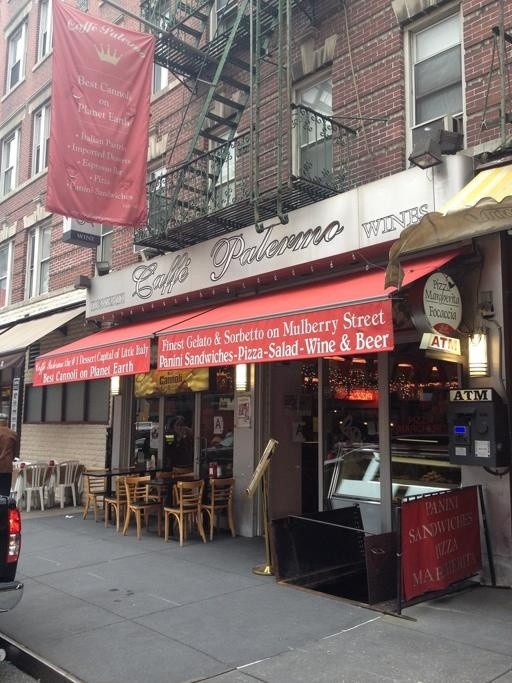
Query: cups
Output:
[[50, 460, 54, 466], [20, 463, 25, 468]]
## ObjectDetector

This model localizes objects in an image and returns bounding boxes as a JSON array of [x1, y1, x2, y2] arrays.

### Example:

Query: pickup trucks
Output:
[[0, 495, 24, 612]]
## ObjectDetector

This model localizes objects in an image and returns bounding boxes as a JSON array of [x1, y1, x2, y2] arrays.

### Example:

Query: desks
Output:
[[82, 468, 164, 527], [12, 462, 84, 508], [140, 476, 233, 538]]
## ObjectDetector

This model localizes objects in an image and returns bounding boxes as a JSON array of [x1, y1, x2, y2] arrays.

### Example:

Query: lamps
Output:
[[467, 333, 488, 375], [111, 377, 120, 397], [237, 364, 245, 390]]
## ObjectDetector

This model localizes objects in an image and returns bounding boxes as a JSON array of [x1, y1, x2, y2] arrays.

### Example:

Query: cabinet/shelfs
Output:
[[336, 452, 462, 503]]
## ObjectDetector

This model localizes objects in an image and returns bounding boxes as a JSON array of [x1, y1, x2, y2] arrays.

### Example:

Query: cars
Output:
[[323, 446, 461, 502]]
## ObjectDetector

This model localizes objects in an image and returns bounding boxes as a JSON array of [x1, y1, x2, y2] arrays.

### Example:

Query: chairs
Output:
[[124, 477, 162, 539], [83, 467, 116, 524], [164, 479, 207, 546], [146, 471, 176, 536], [202, 479, 236, 541], [23, 463, 47, 511], [104, 474, 139, 532], [53, 460, 79, 507]]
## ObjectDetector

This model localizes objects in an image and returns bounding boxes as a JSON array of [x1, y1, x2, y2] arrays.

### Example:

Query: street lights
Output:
[[104, 374, 120, 520]]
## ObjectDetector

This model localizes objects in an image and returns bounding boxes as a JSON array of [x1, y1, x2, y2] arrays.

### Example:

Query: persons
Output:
[[1, 415, 20, 494]]
